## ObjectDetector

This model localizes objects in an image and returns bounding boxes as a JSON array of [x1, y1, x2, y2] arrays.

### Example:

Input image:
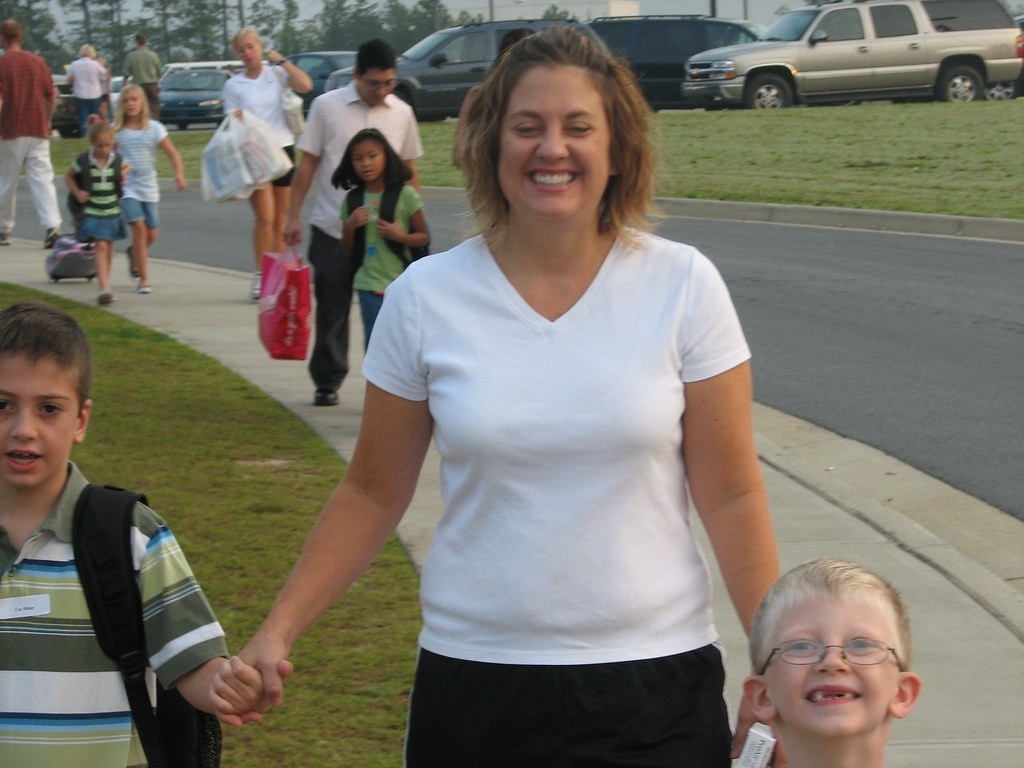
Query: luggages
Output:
[[47, 233, 97, 282]]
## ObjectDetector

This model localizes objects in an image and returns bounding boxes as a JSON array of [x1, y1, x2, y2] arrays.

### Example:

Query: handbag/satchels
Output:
[[200, 111, 294, 203], [258, 242, 310, 361], [282, 88, 305, 132]]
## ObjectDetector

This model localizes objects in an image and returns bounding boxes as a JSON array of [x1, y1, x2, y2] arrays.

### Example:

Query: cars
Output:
[[48, 61, 270, 139], [270, 50, 357, 116]]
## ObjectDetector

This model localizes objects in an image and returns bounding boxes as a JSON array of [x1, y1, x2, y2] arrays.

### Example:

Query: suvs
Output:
[[324, 19, 587, 122], [584, 15, 770, 110], [681, 0, 1024, 110]]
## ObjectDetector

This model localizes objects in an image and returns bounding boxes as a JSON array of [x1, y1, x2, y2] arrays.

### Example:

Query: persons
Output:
[[742, 557, 921, 768], [212, 27, 781, 768], [220, 26, 313, 299], [108, 85, 187, 293], [0, 302, 294, 768], [332, 128, 430, 357], [122, 32, 164, 121], [64, 44, 113, 136], [0, 18, 62, 250], [66, 113, 129, 306], [281, 39, 424, 405]]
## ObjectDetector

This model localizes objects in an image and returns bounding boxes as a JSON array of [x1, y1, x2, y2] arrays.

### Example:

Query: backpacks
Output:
[[72, 483, 223, 768]]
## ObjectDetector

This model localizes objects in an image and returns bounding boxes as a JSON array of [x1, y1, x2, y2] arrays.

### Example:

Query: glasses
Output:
[[757, 637, 903, 674]]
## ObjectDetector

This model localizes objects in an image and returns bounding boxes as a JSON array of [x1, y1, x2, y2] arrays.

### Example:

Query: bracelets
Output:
[[277, 57, 287, 65]]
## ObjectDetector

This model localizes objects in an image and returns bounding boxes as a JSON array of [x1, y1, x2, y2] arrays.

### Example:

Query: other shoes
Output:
[[139, 285, 151, 293], [0, 234, 10, 245], [128, 246, 139, 281], [45, 234, 58, 248], [97, 291, 110, 306], [250, 271, 262, 298], [314, 388, 339, 406]]
